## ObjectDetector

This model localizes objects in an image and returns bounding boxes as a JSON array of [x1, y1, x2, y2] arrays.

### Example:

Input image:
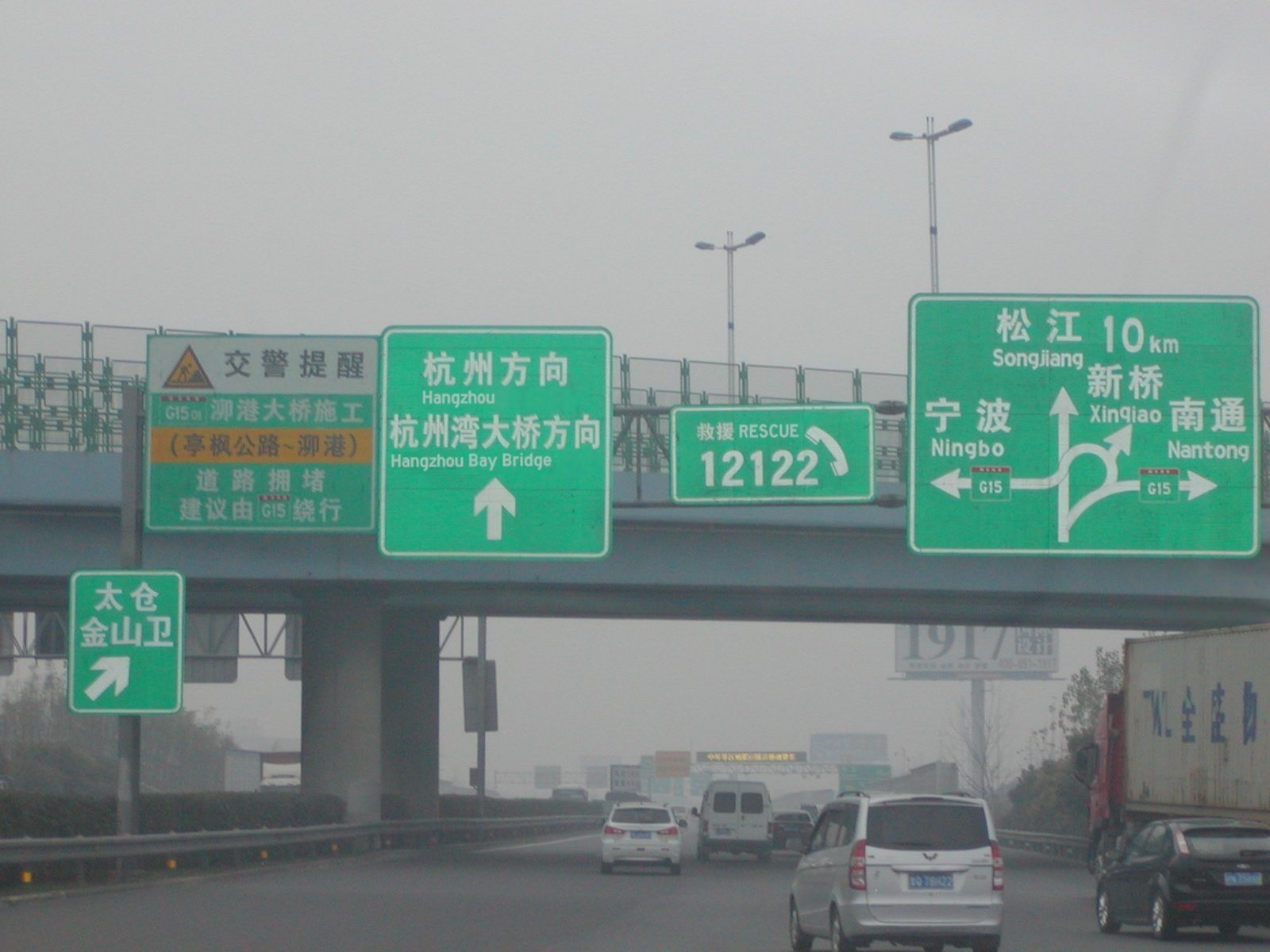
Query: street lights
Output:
[[890, 115, 973, 292], [696, 231, 768, 404]]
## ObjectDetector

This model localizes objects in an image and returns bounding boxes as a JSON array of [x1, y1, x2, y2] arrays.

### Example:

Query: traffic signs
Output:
[[66, 567, 181, 716], [904, 292, 1261, 562], [376, 323, 615, 562]]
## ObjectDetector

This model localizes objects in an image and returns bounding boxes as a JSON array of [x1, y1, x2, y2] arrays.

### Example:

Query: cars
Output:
[[772, 802, 820, 843], [1095, 816, 1269, 942], [598, 800, 689, 877]]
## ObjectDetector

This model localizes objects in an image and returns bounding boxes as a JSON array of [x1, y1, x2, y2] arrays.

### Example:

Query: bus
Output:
[[550, 785, 588, 802]]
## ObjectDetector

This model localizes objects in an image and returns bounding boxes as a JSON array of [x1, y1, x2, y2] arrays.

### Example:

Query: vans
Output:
[[691, 779, 777, 863], [786, 788, 1006, 952]]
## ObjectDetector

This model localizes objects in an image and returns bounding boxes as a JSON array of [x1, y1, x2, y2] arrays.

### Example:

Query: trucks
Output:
[[1072, 623, 1270, 874]]
